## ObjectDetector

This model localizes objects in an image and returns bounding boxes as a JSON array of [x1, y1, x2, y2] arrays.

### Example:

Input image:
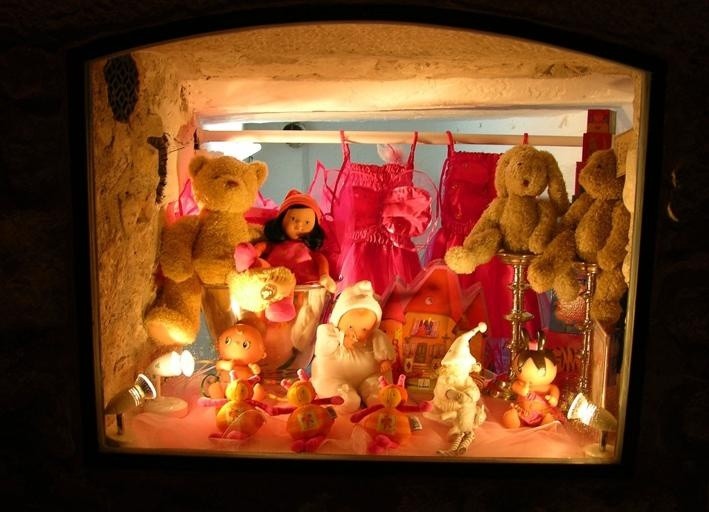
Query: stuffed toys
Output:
[[142, 141, 630, 457], [144, 141, 630, 456]]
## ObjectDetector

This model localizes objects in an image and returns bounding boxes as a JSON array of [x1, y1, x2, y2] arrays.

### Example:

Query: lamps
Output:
[[103, 348, 196, 444], [566, 391, 618, 459]]
